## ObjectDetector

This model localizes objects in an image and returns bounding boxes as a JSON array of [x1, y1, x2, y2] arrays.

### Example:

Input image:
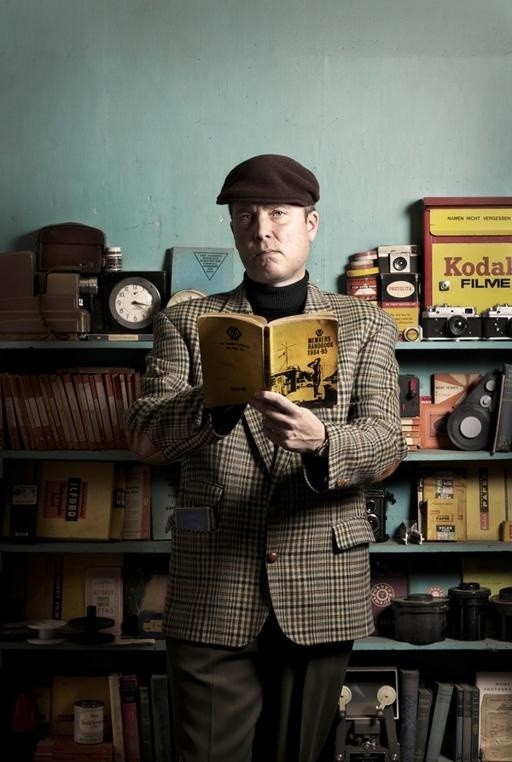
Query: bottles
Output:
[[388, 581, 512, 646], [105, 246, 123, 272]]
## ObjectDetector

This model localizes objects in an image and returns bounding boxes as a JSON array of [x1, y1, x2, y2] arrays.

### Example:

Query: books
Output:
[[399, 670, 512, 761], [35, 735, 113, 760], [2, 372, 142, 450], [196, 313, 340, 411], [121, 553, 170, 640], [401, 373, 512, 543], [84, 567, 123, 636], [10, 460, 176, 540], [108, 672, 175, 760]]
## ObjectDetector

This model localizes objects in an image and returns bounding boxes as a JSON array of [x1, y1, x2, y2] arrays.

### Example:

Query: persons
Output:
[[308, 358, 322, 399], [125, 153, 404, 761]]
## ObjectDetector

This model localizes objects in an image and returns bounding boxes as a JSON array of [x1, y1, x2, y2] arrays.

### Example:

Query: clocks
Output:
[[98, 270, 170, 335]]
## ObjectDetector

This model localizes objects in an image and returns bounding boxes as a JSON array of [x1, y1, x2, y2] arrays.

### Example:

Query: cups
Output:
[[74, 701, 104, 744]]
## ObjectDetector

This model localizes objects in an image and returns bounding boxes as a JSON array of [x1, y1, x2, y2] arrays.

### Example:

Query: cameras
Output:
[[378, 244, 418, 273], [422, 303, 482, 341], [482, 303, 512, 341]]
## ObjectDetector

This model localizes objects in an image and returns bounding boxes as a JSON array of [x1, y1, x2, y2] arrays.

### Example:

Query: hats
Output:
[[214, 154, 321, 208]]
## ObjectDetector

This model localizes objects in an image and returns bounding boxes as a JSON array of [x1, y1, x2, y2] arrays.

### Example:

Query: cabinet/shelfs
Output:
[[2, 338, 511, 762]]
